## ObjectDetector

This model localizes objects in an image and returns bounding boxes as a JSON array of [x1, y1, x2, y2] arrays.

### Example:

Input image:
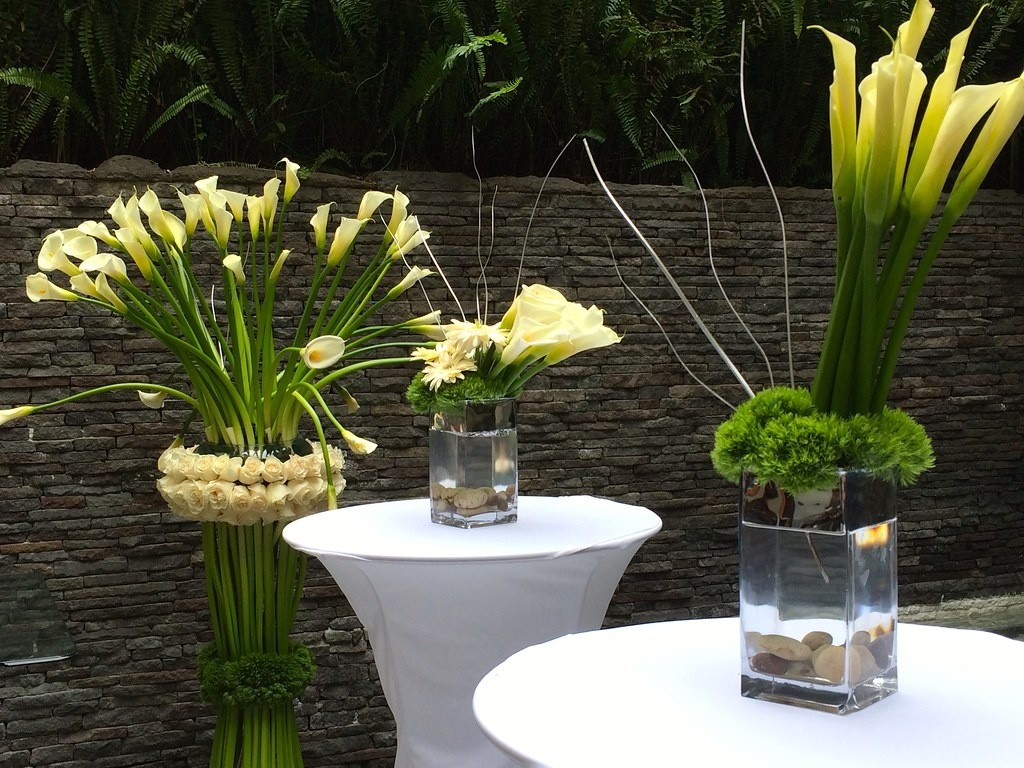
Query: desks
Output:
[[282, 495, 662, 767], [472, 618, 1024, 768]]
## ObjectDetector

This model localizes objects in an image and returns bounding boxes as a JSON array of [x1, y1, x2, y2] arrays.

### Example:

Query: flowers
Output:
[[406, 283, 624, 404], [1, 156, 418, 767], [712, 0, 1024, 495]]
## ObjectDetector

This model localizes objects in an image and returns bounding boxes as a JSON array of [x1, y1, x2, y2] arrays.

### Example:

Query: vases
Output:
[[429, 398, 518, 529], [739, 467, 897, 715], [194, 438, 313, 463]]
